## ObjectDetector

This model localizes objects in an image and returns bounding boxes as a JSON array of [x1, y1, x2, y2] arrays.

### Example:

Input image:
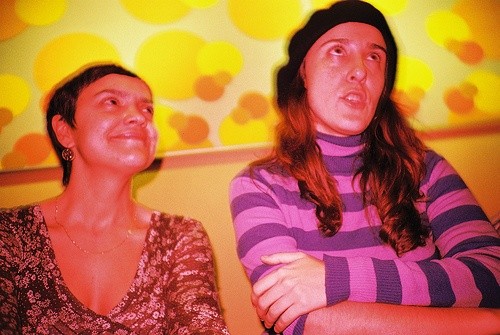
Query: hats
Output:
[[284, 0, 397, 72]]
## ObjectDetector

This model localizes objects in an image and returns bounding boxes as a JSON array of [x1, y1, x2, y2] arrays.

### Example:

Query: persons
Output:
[[229, 0, 500, 335], [0, 60, 227, 335]]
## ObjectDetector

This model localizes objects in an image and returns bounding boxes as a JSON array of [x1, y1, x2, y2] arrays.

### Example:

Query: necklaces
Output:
[[55, 195, 136, 253]]
